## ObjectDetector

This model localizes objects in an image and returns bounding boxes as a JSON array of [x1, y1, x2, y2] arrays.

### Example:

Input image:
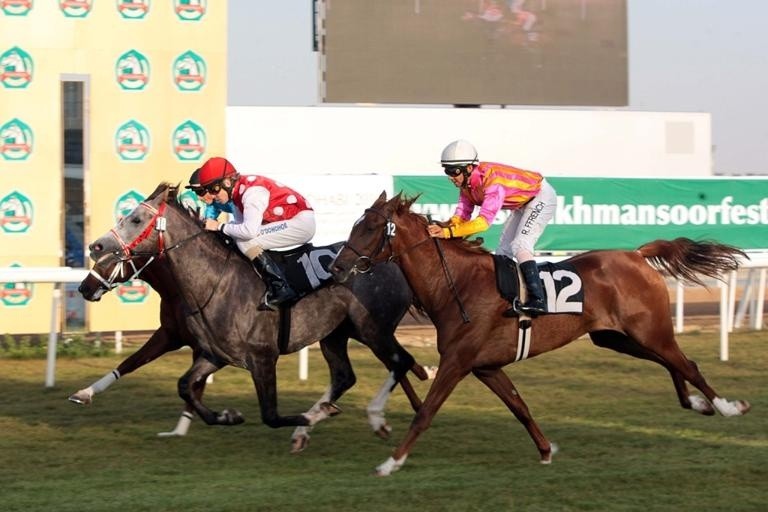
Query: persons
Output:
[[185, 168, 236, 223], [199, 157, 316, 311], [426, 140, 558, 318]]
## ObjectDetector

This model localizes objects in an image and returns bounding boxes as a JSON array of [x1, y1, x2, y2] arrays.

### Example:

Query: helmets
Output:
[[185, 157, 237, 191], [436, 140, 480, 165]]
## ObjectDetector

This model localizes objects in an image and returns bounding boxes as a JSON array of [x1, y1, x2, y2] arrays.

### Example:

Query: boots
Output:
[[519, 260, 545, 315], [252, 251, 298, 310]]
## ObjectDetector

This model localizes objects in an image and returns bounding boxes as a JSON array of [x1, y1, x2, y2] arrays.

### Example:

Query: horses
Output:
[[326, 187, 752, 477], [68, 180, 442, 454]]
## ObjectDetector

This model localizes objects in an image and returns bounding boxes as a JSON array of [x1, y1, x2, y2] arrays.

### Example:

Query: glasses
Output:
[[444, 165, 463, 176], [195, 179, 223, 196]]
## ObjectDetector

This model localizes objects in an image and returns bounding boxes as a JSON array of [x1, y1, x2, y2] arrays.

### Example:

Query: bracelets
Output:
[[447, 227, 454, 238], [220, 224, 225, 232]]
